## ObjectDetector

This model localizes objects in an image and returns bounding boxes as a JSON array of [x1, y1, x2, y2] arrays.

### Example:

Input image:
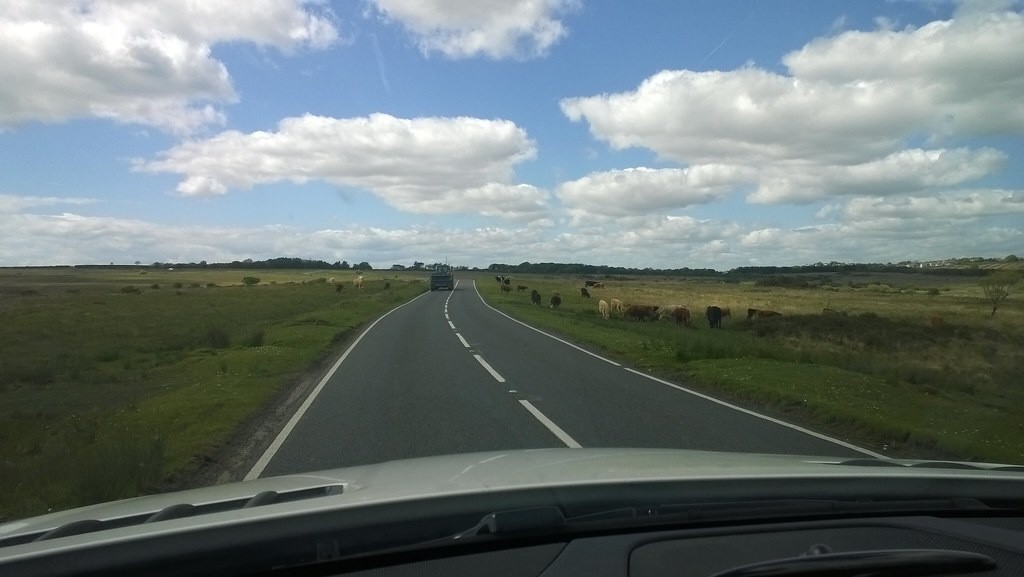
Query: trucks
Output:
[[431, 263, 454, 291]]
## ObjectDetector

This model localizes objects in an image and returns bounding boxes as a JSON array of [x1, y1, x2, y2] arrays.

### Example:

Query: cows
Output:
[[384, 281, 391, 291], [328, 277, 345, 294], [496, 274, 785, 329], [352, 275, 365, 289]]
